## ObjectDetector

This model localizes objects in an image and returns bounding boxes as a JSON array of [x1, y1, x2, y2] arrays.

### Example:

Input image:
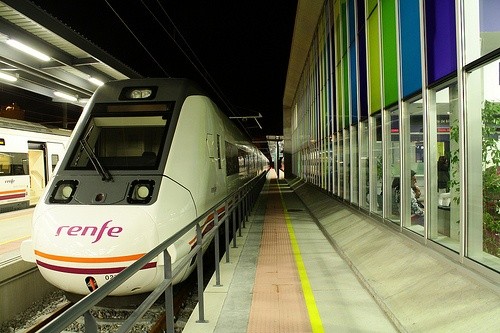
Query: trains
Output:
[[32, 77, 271, 298], [1, 118, 74, 206]]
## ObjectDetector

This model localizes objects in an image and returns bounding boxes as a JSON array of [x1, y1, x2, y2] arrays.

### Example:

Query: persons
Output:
[[399, 169, 425, 217], [413, 186, 425, 209], [377, 191, 383, 210], [392, 178, 400, 215], [438, 156, 450, 192]]
